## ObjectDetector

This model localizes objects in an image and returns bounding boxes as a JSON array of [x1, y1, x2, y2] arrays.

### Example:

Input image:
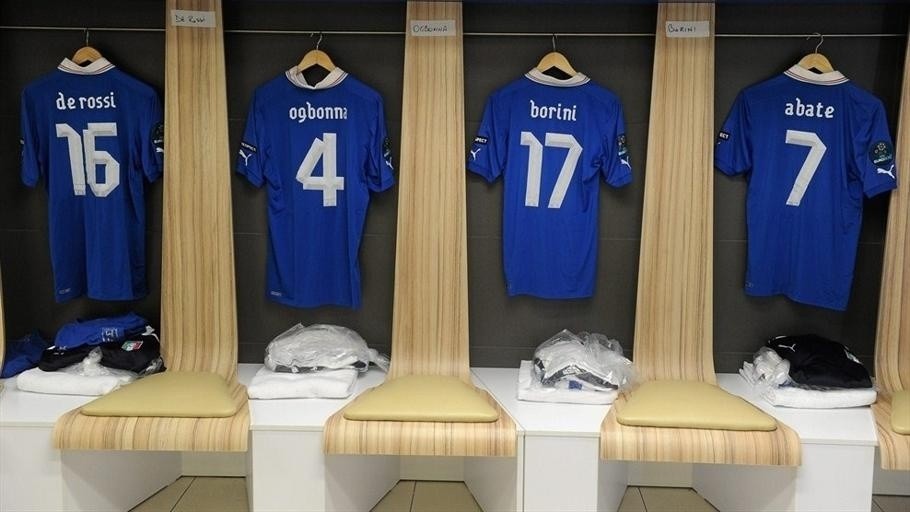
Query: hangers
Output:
[[537, 32, 577, 76], [297, 30, 335, 73], [797, 32, 834, 73], [72, 29, 101, 65]]
